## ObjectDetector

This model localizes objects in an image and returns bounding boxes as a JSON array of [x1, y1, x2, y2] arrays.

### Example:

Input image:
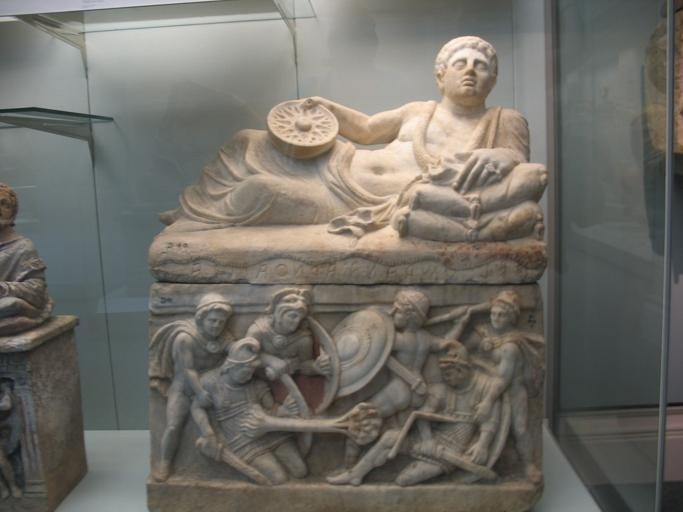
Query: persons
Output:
[[156, 35, 531, 233], [0, 183, 54, 337], [150, 290, 551, 481]]
